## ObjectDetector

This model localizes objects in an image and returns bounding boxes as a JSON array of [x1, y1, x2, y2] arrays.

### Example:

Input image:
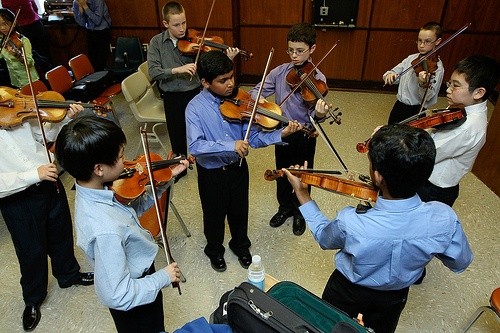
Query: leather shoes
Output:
[[22, 290, 48, 332], [293, 210, 306, 236], [237, 248, 253, 269], [60, 272, 94, 289], [269, 210, 293, 227], [210, 255, 227, 272]]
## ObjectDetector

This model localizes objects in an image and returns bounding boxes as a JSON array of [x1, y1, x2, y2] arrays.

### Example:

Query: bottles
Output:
[[247, 255, 266, 291]]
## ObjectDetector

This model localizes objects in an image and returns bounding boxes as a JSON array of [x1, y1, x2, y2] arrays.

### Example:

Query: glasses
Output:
[[285, 46, 311, 56]]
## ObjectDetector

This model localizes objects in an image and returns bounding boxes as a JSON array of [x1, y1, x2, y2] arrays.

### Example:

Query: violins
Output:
[[356, 105, 467, 153], [264, 168, 379, 203], [177, 28, 254, 62], [285, 61, 342, 125], [0, 85, 113, 127], [107, 151, 195, 205], [411, 51, 439, 88], [219, 87, 320, 139], [2, 30, 35, 68]]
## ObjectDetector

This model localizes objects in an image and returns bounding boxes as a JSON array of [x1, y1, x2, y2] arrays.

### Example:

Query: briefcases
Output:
[[211, 282, 376, 333]]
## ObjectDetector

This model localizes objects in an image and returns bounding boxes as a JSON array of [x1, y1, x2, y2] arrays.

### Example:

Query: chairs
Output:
[[21, 36, 192, 282]]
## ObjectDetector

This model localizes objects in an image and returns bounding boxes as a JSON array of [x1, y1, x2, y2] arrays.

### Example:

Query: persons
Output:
[[246, 21, 328, 235], [56, 116, 189, 333], [148, 1, 240, 171], [282, 124, 472, 333], [356, 52, 497, 210], [0, 72, 94, 331], [0, 8, 47, 97], [72, 0, 113, 69], [381, 22, 446, 129], [185, 50, 302, 272]]
